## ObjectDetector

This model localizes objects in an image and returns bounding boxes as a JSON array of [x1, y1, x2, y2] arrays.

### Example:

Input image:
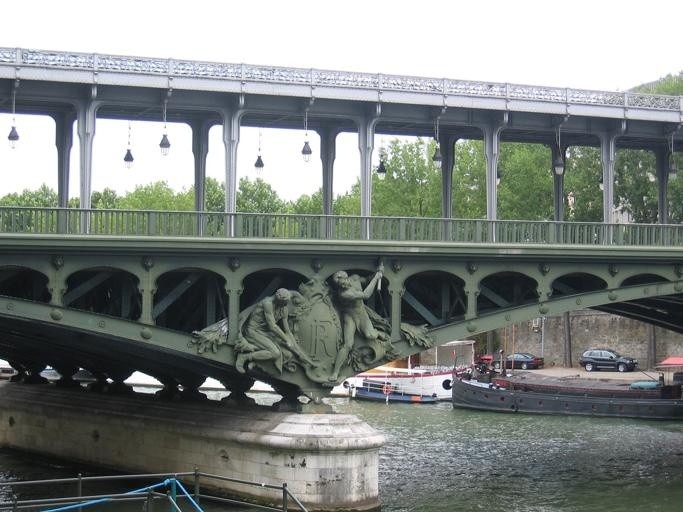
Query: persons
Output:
[[236, 288, 298, 374], [328, 265, 385, 381]]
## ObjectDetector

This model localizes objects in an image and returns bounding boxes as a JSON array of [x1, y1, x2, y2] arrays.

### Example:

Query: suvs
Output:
[[578, 347, 638, 372]]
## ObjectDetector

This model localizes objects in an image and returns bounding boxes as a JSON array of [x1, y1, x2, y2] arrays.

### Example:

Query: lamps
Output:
[[299, 111, 315, 163], [122, 115, 136, 170], [158, 101, 173, 156], [496, 172, 501, 186], [431, 118, 444, 169], [8, 90, 21, 150], [375, 128, 388, 182], [253, 121, 266, 175], [552, 155, 565, 176]]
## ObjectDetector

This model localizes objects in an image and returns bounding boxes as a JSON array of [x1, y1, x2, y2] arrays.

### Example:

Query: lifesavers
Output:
[[384, 384, 393, 394]]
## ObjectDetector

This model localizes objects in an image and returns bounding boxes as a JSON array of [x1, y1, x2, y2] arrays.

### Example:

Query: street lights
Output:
[[498, 349, 504, 374]]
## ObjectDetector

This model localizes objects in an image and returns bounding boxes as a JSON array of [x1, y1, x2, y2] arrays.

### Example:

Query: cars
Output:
[[492, 351, 545, 370]]
